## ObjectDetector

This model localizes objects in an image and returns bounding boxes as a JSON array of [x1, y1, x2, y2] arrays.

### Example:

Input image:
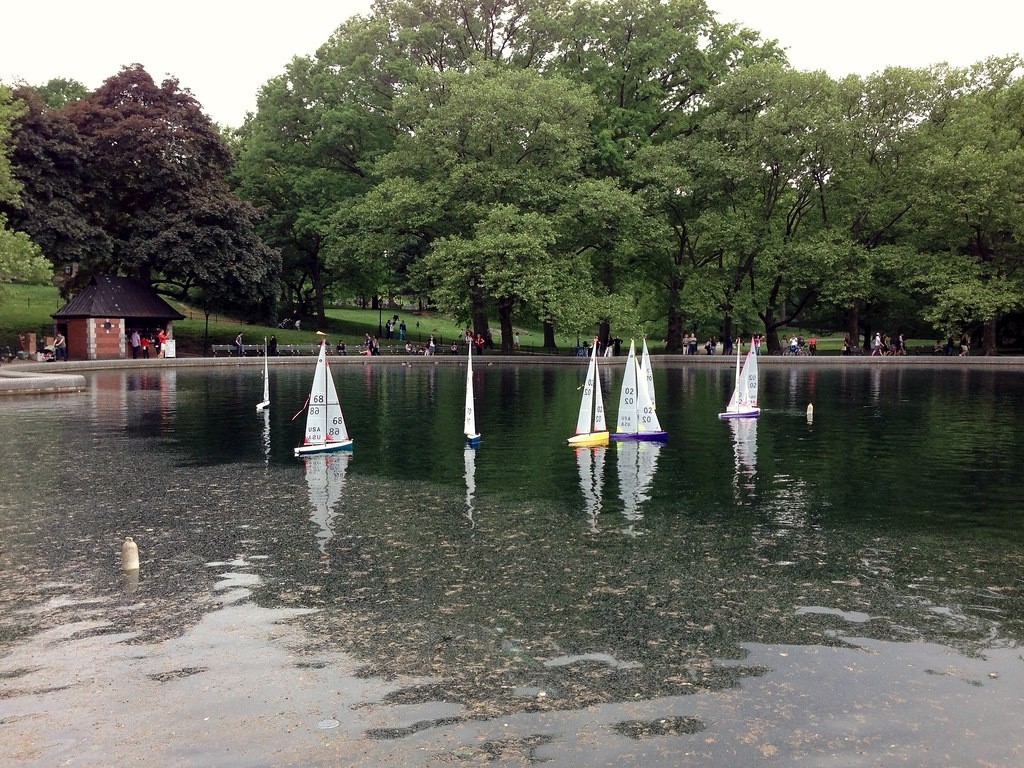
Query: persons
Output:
[[844, 334, 851, 355], [451, 340, 458, 355], [590, 332, 624, 356], [53, 331, 68, 361], [336, 340, 346, 356], [514, 332, 521, 352], [359, 332, 381, 355], [428, 334, 437, 356], [780, 334, 816, 354], [681, 331, 764, 355], [933, 334, 970, 356], [871, 332, 906, 356], [268, 335, 277, 356], [236, 331, 246, 357], [404, 341, 429, 356], [385, 320, 406, 341], [465, 329, 485, 356], [131, 327, 172, 359]]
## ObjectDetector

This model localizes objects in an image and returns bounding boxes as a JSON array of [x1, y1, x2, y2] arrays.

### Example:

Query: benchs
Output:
[[915, 346, 945, 355], [436, 346, 453, 355], [211, 344, 406, 357]]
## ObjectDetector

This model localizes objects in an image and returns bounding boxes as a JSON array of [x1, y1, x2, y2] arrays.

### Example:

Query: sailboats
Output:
[[293, 339, 353, 453], [718, 336, 760, 418], [609, 337, 668, 441], [567, 336, 609, 443], [256, 337, 270, 408], [463, 339, 481, 443]]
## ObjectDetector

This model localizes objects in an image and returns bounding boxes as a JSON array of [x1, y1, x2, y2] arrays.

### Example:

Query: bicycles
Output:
[[773, 346, 821, 356]]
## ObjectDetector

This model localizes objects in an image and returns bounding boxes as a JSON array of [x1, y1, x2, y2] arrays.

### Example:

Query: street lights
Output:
[[735, 319, 738, 338], [203, 306, 212, 357], [377, 299, 383, 339]]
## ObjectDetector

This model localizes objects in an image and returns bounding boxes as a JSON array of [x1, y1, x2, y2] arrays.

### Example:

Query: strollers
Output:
[[42, 345, 59, 362]]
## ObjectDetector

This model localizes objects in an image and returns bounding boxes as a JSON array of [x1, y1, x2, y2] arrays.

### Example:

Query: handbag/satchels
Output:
[[841, 345, 847, 350], [143, 346, 146, 350], [232, 338, 239, 346]]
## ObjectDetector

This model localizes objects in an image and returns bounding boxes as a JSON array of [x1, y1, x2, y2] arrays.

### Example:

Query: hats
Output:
[[876, 333, 879, 335]]
